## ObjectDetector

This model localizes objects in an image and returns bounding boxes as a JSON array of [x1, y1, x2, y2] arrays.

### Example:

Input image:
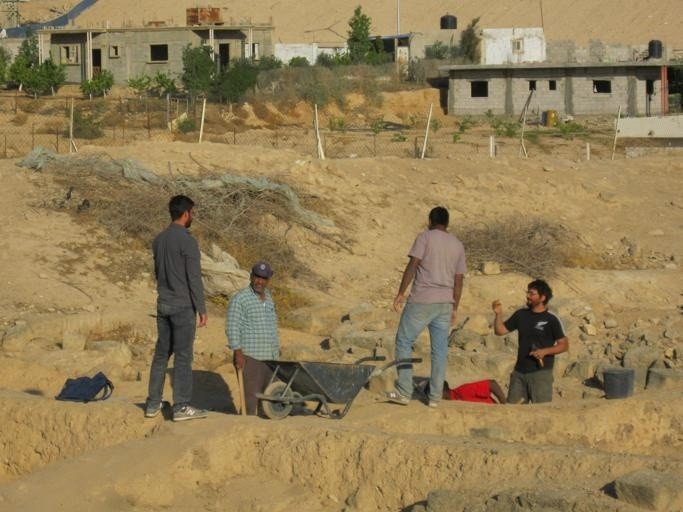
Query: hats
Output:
[[252, 262, 272, 279]]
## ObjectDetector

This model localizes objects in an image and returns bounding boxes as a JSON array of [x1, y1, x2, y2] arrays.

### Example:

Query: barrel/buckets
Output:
[[603, 369, 635, 398]]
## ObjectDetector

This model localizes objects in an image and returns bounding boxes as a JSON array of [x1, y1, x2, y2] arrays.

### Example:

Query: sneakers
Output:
[[146, 401, 166, 417], [378, 390, 410, 406], [173, 406, 209, 422], [424, 396, 439, 408]]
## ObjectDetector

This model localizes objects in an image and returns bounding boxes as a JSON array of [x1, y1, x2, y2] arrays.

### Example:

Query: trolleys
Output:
[[251, 353, 423, 422]]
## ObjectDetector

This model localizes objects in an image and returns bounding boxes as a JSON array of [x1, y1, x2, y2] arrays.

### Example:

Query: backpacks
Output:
[[54, 371, 114, 403]]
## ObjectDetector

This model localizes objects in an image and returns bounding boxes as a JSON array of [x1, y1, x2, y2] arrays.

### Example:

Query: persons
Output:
[[375, 207, 468, 408], [145, 195, 209, 423], [492, 280, 571, 405], [227, 264, 283, 421]]
